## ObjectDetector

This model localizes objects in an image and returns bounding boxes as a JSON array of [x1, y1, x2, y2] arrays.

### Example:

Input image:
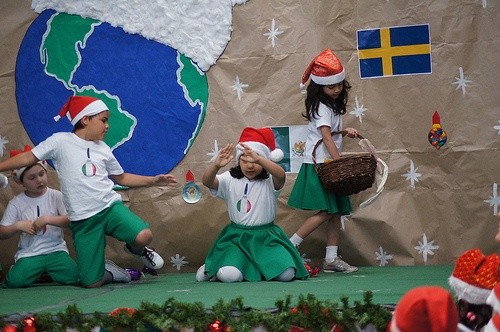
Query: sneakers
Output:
[[123, 242, 165, 270], [104, 259, 132, 283], [322, 255, 359, 273]]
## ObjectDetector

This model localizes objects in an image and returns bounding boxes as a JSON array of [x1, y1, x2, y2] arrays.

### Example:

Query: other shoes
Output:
[[195, 263, 209, 282]]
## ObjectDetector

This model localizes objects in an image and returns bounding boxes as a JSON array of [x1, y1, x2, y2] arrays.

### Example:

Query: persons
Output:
[[0, 95, 177, 287], [0, 144, 78, 288], [196, 127, 310, 282], [287, 48, 358, 273], [386, 248, 500, 332]]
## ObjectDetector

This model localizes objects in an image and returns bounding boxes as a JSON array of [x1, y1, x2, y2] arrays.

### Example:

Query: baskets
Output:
[[312, 129, 377, 196]]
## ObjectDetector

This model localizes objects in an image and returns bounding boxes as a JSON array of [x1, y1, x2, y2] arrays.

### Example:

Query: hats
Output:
[[386, 285, 460, 331], [299, 47, 345, 90], [235, 126, 284, 165], [449, 248, 500, 305], [53, 96, 109, 126], [9, 144, 48, 183]]
